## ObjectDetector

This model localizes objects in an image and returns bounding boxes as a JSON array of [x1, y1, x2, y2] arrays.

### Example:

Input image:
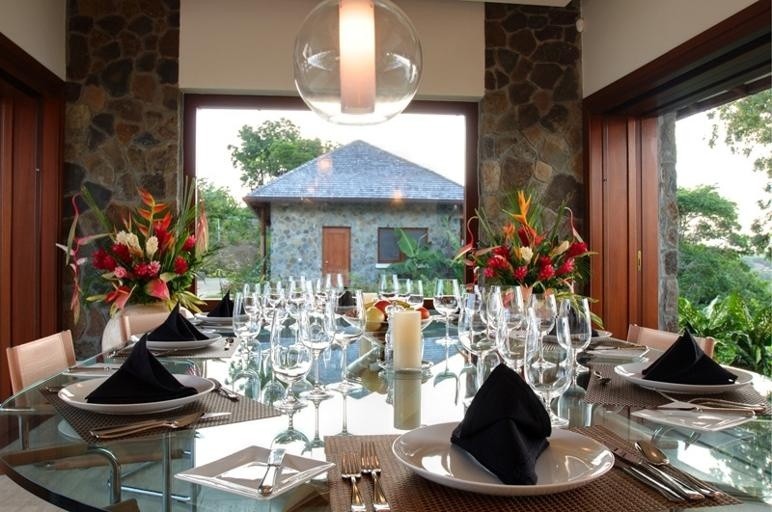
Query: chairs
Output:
[[8, 329, 78, 397], [578, 324, 717, 448], [100, 303, 173, 353]]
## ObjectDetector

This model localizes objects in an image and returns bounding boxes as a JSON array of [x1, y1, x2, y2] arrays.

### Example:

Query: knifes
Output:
[[257, 447, 286, 496], [645, 406, 766, 411], [603, 440, 704, 498], [88, 411, 231, 437]]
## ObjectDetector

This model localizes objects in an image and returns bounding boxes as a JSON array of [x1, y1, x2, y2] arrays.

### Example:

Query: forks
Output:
[[343, 440, 390, 511]]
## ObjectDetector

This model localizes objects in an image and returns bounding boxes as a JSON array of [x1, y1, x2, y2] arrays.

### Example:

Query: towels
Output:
[[339, 291, 357, 306]]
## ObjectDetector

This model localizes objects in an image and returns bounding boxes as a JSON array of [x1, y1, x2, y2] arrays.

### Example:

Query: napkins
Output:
[[547, 306, 599, 336], [338, 287, 354, 305], [86, 333, 191, 401], [151, 304, 204, 340]]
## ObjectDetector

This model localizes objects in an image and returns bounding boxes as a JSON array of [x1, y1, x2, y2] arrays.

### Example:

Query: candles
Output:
[[393, 371, 421, 431], [391, 311, 421, 371]]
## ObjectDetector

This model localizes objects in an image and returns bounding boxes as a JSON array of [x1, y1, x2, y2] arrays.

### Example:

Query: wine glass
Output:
[[270, 389, 354, 460], [433, 278, 592, 425], [233, 274, 367, 408]]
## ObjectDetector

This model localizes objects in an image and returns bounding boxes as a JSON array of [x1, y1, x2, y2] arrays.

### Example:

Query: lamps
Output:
[[292, 0, 424, 126]]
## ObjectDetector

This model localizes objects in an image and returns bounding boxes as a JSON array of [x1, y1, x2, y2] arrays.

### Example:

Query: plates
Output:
[[174, 444, 336, 502], [58, 312, 249, 415], [392, 420, 618, 497], [592, 329, 756, 433]]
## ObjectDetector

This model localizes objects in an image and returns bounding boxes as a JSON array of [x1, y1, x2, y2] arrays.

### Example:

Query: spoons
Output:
[[91, 411, 205, 439], [635, 440, 722, 498]]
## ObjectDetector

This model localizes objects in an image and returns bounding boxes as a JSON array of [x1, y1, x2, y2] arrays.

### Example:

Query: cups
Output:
[[379, 273, 424, 309]]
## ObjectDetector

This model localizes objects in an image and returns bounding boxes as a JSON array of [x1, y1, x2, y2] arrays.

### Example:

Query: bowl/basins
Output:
[[344, 314, 432, 347]]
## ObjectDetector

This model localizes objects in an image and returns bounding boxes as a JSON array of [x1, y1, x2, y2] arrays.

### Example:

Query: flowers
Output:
[[55, 156, 226, 325], [450, 187, 606, 331]]
